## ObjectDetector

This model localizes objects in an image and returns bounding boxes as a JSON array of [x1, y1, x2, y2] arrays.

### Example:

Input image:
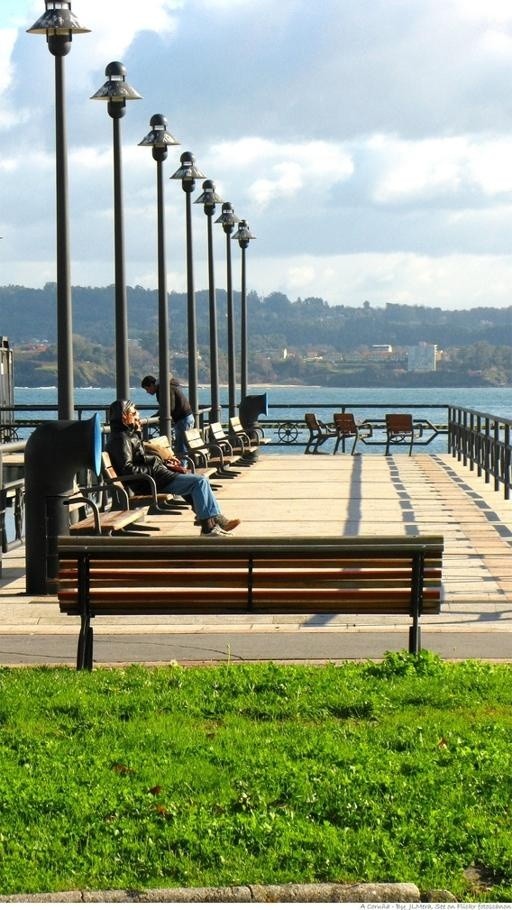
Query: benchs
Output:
[[57, 526, 449, 674], [57, 409, 269, 535]]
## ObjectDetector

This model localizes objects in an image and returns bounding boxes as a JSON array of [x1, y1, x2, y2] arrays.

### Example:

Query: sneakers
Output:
[[200, 524, 234, 537], [215, 515, 239, 531]]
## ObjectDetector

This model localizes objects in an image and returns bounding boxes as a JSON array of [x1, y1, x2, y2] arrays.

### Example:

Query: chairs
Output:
[[384, 413, 425, 457], [302, 413, 338, 454], [334, 411, 373, 457]]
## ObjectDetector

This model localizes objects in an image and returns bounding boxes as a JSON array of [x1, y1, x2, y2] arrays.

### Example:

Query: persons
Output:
[[107, 400, 242, 538], [141, 375, 196, 470]]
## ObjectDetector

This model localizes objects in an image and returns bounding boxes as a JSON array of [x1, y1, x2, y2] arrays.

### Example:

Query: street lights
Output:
[[25, 1, 91, 422], [140, 109, 179, 440], [213, 201, 243, 420], [91, 55, 142, 419], [230, 220, 257, 403], [167, 151, 211, 434], [191, 179, 225, 436]]
